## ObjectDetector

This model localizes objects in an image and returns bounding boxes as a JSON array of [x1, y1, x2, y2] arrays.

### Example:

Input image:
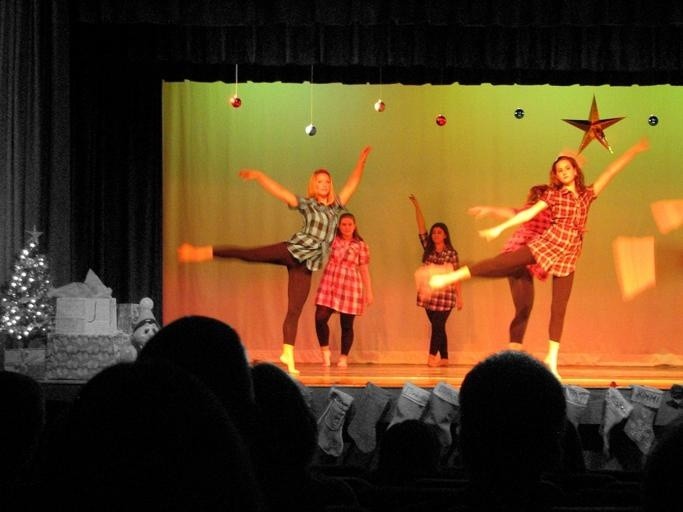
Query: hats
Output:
[[132, 296, 156, 327]]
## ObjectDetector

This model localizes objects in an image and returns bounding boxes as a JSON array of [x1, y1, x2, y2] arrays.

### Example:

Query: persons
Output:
[[428, 142, 653, 382], [314, 213, 374, 369], [177, 146, 368, 374], [407, 192, 463, 367], [467, 184, 554, 353]]
